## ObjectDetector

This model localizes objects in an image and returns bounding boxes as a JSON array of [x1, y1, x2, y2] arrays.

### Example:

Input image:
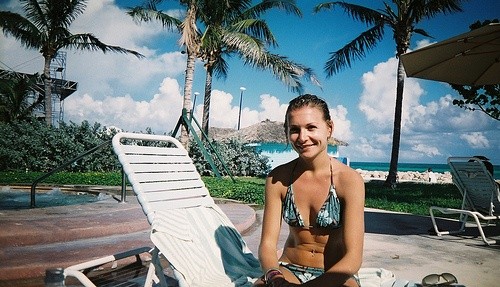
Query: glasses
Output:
[[422, 273, 458, 287]]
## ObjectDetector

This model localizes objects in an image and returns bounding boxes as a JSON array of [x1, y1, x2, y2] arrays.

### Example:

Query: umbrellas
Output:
[[402, 20, 500, 87]]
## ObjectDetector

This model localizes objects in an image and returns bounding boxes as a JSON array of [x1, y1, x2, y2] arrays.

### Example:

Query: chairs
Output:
[[429, 157, 500, 245], [62, 132, 418, 287]]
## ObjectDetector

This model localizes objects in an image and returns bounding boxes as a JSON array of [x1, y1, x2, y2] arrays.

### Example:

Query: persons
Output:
[[254, 94, 365, 287], [370, 167, 448, 183]]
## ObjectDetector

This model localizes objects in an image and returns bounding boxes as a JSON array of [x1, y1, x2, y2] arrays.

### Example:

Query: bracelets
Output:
[[264, 267, 283, 285]]
